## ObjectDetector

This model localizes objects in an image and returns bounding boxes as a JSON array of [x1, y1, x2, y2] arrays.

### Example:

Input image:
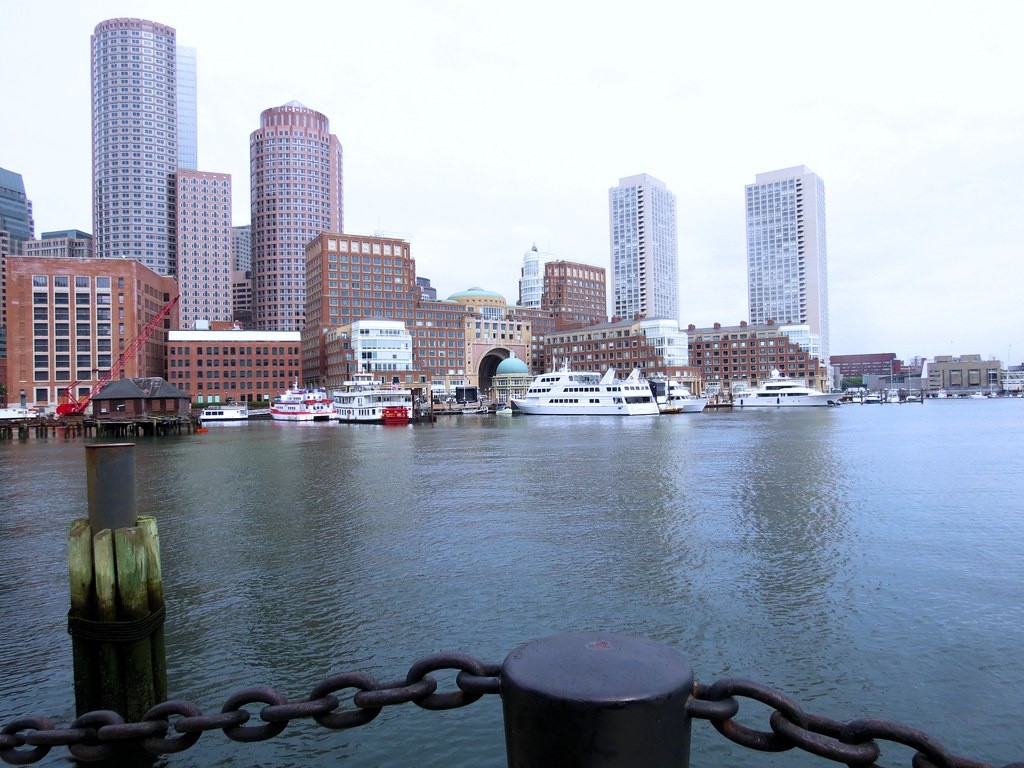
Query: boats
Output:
[[733, 368, 845, 406], [332, 367, 413, 423], [853, 391, 885, 402], [970, 391, 988, 399], [198, 401, 248, 420], [266, 376, 337, 421], [496, 355, 706, 417]]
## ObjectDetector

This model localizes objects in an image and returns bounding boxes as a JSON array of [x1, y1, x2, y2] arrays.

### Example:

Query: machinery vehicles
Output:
[[55, 295, 181, 416]]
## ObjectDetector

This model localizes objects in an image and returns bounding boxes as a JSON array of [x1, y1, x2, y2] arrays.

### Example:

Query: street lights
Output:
[[226, 391, 227, 404]]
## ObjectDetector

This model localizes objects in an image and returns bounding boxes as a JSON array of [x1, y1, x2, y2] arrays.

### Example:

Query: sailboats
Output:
[[886, 358, 918, 403]]
